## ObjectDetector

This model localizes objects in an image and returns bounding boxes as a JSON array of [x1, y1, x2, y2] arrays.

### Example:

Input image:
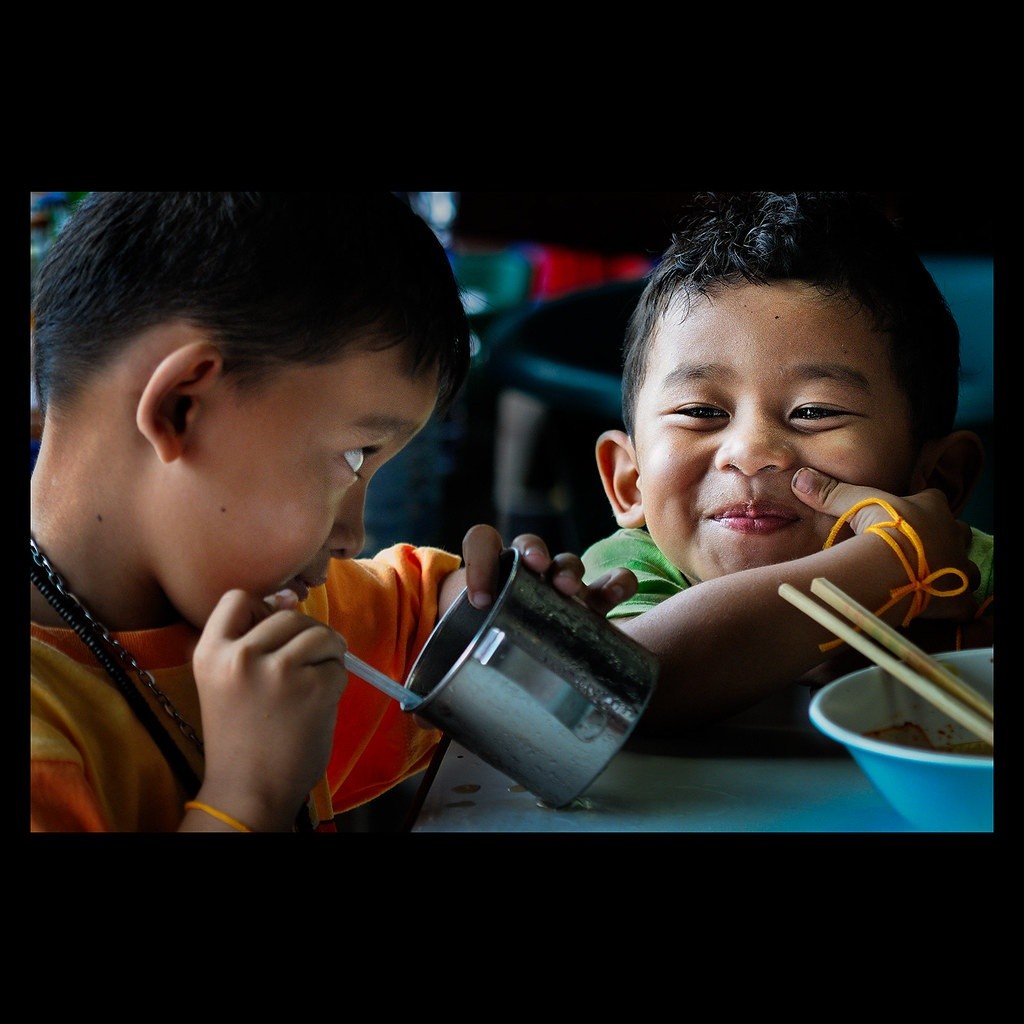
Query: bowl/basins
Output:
[[809, 648, 994, 832]]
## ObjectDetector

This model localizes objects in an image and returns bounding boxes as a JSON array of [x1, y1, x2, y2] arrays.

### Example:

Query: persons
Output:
[[30, 190, 636, 834], [579, 190, 996, 727]]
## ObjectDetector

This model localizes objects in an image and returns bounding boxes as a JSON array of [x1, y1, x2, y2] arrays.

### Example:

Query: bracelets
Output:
[[818, 497, 968, 653], [183, 802, 250, 832]]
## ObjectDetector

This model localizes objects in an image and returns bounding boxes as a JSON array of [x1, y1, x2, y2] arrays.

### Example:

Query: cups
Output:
[[400, 545, 658, 811]]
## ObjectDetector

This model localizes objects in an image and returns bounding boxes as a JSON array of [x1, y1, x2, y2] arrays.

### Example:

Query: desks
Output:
[[407, 729, 928, 833]]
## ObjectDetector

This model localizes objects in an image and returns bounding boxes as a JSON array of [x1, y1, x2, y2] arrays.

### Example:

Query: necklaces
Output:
[[31, 538, 207, 793]]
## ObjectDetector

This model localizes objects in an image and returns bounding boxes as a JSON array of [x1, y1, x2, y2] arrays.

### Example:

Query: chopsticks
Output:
[[777, 577, 994, 746]]
